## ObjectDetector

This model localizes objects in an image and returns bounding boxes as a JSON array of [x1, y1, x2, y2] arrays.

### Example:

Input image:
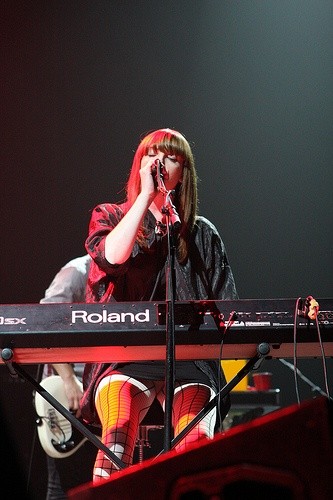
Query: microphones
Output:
[[151, 159, 164, 179]]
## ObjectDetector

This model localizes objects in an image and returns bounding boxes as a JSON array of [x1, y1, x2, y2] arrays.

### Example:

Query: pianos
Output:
[[0, 299, 333, 366]]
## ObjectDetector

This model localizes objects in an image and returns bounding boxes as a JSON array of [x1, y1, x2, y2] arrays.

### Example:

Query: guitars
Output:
[[35, 374, 88, 459]]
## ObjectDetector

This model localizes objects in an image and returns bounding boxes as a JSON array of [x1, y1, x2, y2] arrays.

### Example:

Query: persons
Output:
[[82, 124, 248, 485], [31, 252, 94, 500]]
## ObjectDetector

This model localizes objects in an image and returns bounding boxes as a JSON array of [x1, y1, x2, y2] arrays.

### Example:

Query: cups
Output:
[[253, 372, 272, 390]]
